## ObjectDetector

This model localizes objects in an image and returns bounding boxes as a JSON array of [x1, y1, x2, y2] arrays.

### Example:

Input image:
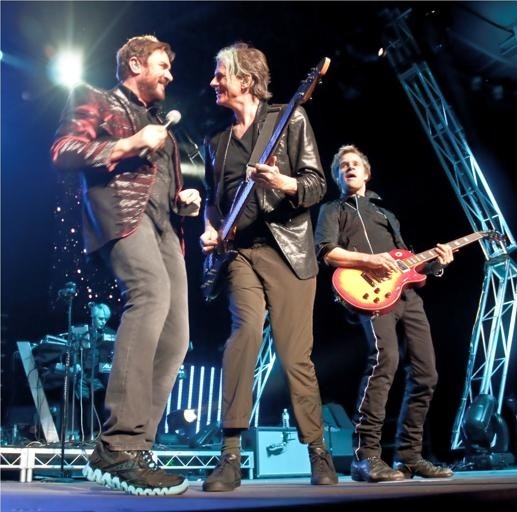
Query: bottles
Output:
[[282, 408, 289, 428], [11, 424, 19, 445]]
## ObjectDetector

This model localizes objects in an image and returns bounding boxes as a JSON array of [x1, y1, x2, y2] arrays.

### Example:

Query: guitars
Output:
[[330, 229, 506, 316], [201, 56, 330, 306]]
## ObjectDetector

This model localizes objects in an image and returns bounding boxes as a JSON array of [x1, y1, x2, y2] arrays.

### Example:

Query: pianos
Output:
[[83, 333, 194, 379]]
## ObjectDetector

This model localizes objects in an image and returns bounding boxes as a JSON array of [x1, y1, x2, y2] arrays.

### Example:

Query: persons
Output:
[[314, 144, 455, 483], [75, 303, 116, 441], [198, 43, 339, 492], [48, 33, 203, 498]]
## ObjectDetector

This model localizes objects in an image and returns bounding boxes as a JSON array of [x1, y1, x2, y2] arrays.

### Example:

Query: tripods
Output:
[[39, 342, 96, 449]]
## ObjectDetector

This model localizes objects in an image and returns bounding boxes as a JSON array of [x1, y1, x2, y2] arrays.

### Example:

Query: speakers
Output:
[[319, 402, 356, 474]]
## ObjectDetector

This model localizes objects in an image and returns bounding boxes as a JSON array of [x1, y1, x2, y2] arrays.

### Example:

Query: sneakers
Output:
[[82, 442, 189, 496], [203, 457, 241, 491], [310, 452, 337, 484]]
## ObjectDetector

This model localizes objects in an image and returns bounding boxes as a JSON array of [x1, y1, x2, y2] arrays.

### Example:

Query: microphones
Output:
[[140, 109, 182, 160]]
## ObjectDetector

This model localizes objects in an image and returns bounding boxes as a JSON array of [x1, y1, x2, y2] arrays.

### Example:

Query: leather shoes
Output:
[[351, 456, 453, 482]]
